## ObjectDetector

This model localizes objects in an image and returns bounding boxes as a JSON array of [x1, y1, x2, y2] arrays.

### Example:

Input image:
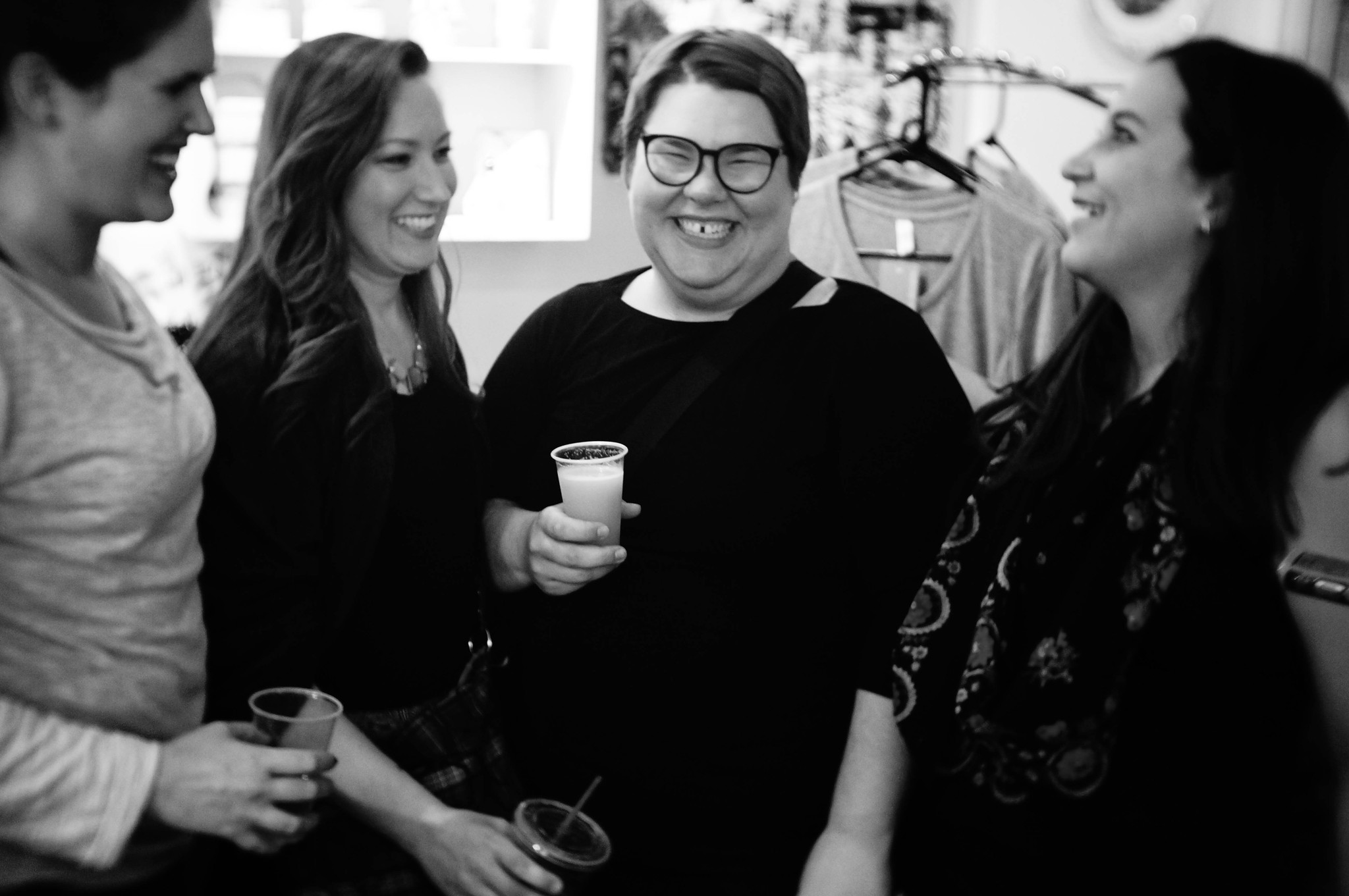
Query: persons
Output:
[[475, 22, 977, 896], [1, 0, 340, 896], [178, 30, 566, 895], [795, 33, 1349, 896]]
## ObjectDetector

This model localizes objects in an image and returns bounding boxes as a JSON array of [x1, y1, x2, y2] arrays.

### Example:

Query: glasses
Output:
[[637, 129, 785, 196]]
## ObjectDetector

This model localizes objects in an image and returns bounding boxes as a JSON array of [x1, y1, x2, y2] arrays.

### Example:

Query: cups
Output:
[[247, 687, 344, 818], [506, 798, 610, 896], [551, 443, 628, 546]]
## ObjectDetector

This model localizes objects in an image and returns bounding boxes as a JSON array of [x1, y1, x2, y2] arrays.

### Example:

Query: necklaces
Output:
[[377, 290, 428, 393]]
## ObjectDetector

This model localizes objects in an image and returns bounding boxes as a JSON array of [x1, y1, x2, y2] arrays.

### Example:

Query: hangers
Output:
[[833, 54, 1023, 205]]
